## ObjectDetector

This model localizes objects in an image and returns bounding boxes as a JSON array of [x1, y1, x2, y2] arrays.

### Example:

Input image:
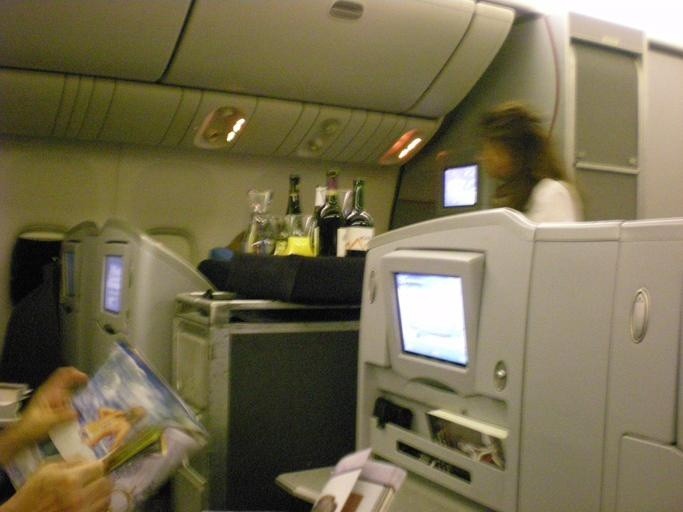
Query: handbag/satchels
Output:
[[1, 281, 65, 407]]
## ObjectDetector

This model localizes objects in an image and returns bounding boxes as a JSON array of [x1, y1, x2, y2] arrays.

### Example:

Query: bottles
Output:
[[317, 170, 345, 258], [344, 177, 373, 258], [245, 186, 281, 254], [305, 184, 326, 256], [284, 174, 303, 234]]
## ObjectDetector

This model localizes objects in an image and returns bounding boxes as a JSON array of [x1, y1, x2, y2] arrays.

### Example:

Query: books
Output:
[[421, 404, 509, 471], [308, 445, 412, 511], [3, 334, 215, 511]]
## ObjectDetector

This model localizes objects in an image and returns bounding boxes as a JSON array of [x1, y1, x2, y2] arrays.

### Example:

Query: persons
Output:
[[0, 364, 114, 511], [470, 100, 584, 225], [78, 403, 148, 450]]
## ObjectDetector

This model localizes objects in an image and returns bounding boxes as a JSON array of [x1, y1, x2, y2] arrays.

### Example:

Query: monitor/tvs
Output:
[[381, 248, 485, 398], [63, 250, 75, 298], [442, 163, 479, 209], [99, 253, 125, 318]]
